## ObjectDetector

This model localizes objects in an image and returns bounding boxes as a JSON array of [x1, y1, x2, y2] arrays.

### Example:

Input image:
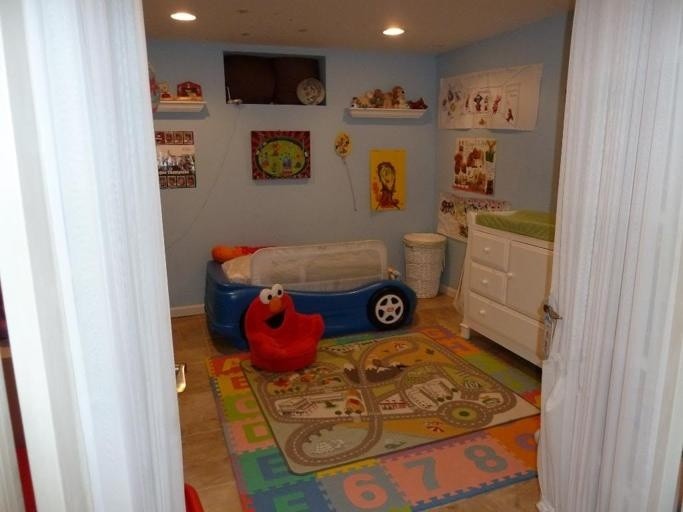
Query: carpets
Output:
[[239, 330, 541, 476], [207, 323, 542, 512]]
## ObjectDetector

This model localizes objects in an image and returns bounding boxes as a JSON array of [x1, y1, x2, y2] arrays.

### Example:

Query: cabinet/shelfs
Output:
[[461, 226, 554, 370]]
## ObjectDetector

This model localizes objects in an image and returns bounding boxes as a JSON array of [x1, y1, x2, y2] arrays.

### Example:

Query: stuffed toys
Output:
[[351, 85, 428, 110]]
